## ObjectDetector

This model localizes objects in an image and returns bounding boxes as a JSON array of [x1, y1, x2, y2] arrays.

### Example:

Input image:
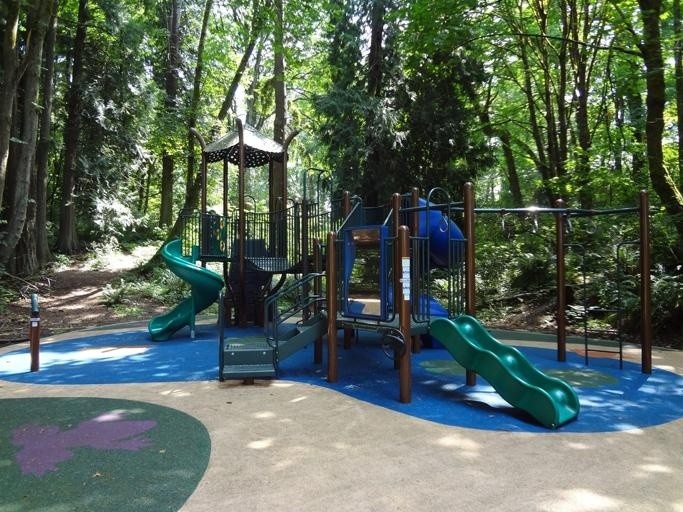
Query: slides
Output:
[[147, 241, 223, 341], [429, 314, 580, 428]]
